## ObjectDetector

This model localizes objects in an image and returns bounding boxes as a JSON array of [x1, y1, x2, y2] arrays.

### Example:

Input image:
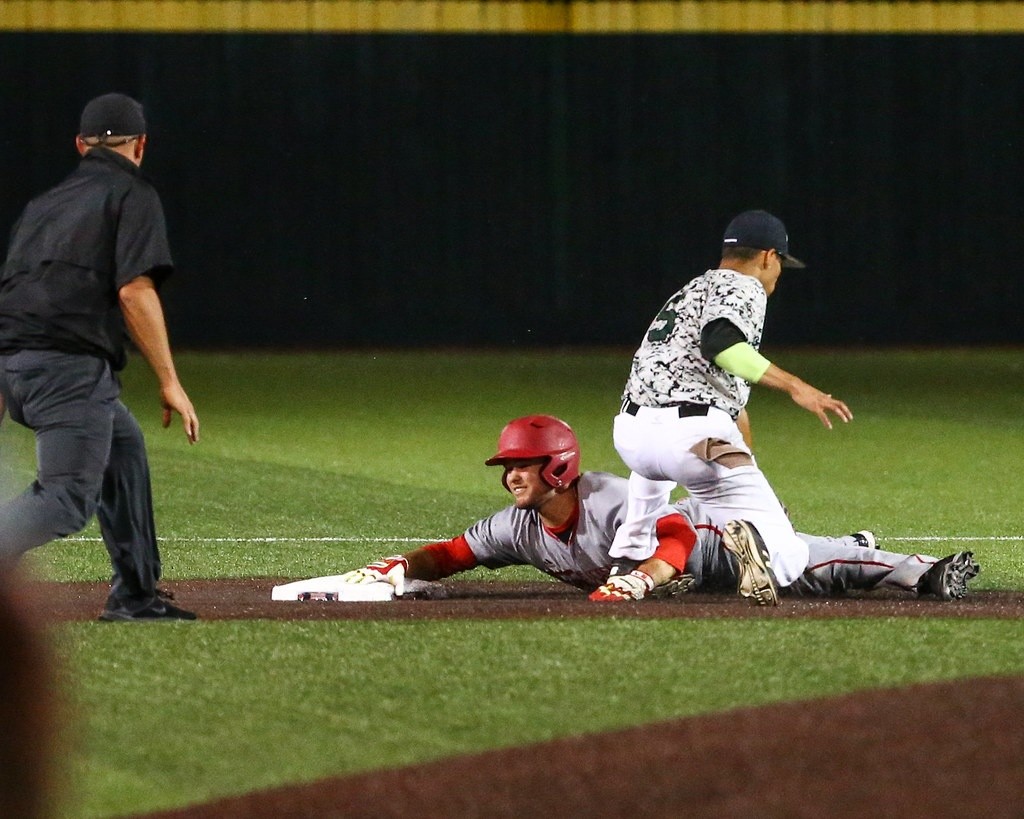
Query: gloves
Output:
[[589, 570, 654, 602], [345, 555, 409, 596]]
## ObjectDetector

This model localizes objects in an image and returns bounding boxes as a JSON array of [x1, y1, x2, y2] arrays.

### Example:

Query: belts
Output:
[[625, 402, 710, 418]]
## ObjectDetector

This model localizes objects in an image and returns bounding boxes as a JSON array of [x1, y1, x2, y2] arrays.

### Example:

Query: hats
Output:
[[722, 210, 806, 268], [80, 93, 146, 137]]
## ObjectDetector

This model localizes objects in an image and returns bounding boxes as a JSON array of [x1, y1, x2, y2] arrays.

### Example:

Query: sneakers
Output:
[[930, 552, 981, 599], [722, 519, 779, 606], [609, 558, 695, 599], [98, 595, 197, 621], [850, 530, 881, 549]]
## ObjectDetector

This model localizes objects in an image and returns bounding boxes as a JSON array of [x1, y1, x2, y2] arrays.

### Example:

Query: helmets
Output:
[[486, 416, 580, 487]]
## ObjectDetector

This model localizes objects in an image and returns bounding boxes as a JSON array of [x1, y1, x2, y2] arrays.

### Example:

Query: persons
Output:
[[609, 211, 854, 603], [346, 414, 981, 605], [0, 91, 199, 622]]
